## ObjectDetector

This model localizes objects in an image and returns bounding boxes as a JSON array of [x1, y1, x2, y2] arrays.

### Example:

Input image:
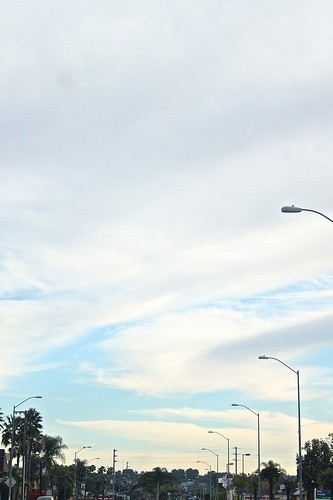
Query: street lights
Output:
[[208, 431, 230, 500], [8, 396, 42, 500], [73, 446, 92, 500], [258, 356, 303, 500], [84, 458, 99, 500], [227, 463, 233, 473], [201, 448, 218, 500], [231, 404, 260, 500], [197, 461, 211, 500], [242, 454, 250, 500]]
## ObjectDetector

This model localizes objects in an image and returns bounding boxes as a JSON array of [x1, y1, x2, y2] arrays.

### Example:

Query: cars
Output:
[[37, 496, 54, 500]]
